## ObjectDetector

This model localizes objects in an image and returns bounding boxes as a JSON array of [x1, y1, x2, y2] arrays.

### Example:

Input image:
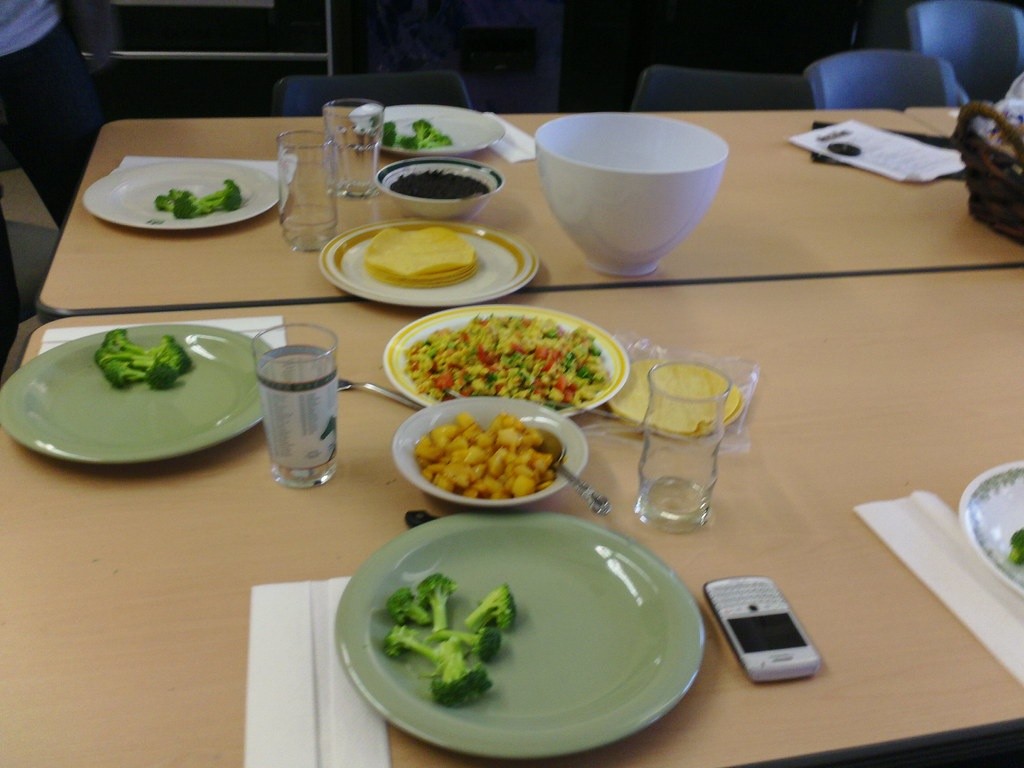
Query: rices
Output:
[[404, 314, 611, 411]]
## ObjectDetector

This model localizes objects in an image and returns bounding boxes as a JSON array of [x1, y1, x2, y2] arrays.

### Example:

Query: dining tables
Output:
[[0, 262, 1024, 768], [0, 108, 1024, 322]]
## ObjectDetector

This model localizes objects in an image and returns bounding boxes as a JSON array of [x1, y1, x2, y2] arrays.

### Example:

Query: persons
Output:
[[0, 0, 101, 352]]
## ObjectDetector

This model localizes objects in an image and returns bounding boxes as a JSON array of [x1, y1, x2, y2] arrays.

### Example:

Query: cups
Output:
[[319, 96, 384, 201], [634, 362, 732, 533], [250, 320, 339, 487], [276, 129, 339, 251]]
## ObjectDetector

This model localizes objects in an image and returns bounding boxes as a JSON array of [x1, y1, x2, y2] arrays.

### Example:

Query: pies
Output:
[[365, 227, 478, 287], [607, 358, 745, 435]]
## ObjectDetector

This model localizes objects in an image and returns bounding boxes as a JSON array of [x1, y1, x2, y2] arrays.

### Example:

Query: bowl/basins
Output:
[[373, 155, 505, 219], [534, 112, 729, 279]]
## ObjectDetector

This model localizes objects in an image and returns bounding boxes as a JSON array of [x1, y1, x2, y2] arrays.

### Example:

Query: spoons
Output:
[[531, 430, 613, 520]]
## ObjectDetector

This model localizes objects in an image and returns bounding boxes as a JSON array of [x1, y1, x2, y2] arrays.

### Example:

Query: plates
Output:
[[84, 163, 283, 230], [350, 105, 508, 155], [0, 322, 271, 465], [332, 508, 706, 757], [390, 398, 589, 508], [961, 459, 1024, 598], [380, 304, 632, 425], [317, 218, 540, 305]]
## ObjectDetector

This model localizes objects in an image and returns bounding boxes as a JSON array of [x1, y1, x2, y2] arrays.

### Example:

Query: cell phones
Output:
[[704, 577, 821, 682]]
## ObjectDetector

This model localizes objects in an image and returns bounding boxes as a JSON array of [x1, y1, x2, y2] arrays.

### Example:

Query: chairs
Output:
[[273, 71, 473, 118], [803, 50, 958, 109], [630, 65, 804, 112], [907, 0, 1024, 104]]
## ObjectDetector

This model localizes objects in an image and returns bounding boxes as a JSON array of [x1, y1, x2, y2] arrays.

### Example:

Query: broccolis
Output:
[[155, 178, 242, 219], [383, 573, 516, 705], [94, 328, 191, 390], [382, 119, 452, 149], [1009, 527, 1024, 564]]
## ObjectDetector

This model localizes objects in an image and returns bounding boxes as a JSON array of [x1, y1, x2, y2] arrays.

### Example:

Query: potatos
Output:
[[414, 412, 556, 500]]
